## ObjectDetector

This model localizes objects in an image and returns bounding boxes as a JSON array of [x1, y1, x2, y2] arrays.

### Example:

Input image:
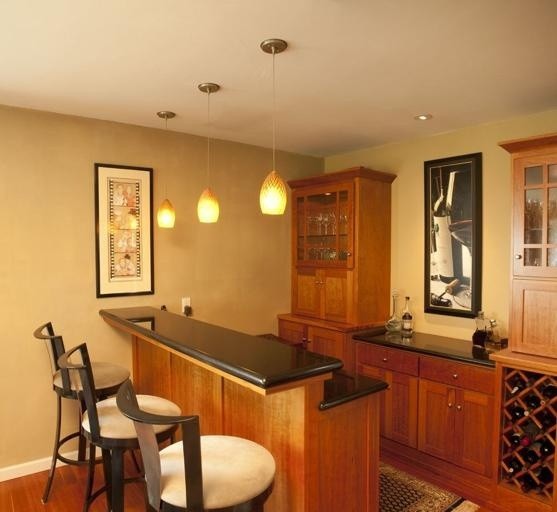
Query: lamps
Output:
[[259, 39, 287, 215], [158, 111, 176, 228], [197, 83, 220, 224]]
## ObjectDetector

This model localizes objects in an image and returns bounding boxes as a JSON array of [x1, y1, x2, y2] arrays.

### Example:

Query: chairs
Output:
[[57, 342, 181, 512], [34, 322, 140, 505], [117, 380, 275, 512]]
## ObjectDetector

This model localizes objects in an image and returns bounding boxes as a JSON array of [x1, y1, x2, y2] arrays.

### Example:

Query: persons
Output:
[[113, 184, 137, 276]]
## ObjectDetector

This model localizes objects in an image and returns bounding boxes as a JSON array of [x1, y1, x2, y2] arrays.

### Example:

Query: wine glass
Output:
[[522, 195, 555, 230], [445, 169, 471, 309], [297, 210, 349, 236]]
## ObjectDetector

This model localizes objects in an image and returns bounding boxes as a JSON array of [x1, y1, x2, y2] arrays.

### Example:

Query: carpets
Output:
[[379, 461, 480, 512]]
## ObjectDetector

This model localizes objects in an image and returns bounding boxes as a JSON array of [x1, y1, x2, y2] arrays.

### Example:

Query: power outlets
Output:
[[182, 297, 190, 313]]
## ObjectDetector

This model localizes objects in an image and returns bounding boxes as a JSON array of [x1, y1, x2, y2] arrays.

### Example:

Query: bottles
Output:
[[399, 296, 413, 336], [432, 167, 462, 283], [381, 289, 403, 332], [302, 247, 347, 260], [505, 371, 556, 497], [474, 310, 488, 332], [485, 319, 502, 343]]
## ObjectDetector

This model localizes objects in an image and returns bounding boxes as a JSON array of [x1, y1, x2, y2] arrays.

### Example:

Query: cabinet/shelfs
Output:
[[353, 340, 420, 477], [418, 353, 502, 509], [287, 166, 397, 327], [278, 319, 386, 376], [498, 133, 557, 360]]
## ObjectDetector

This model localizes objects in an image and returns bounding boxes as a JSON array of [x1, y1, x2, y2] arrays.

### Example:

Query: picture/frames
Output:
[[94, 162, 154, 298], [424, 152, 482, 318]]
